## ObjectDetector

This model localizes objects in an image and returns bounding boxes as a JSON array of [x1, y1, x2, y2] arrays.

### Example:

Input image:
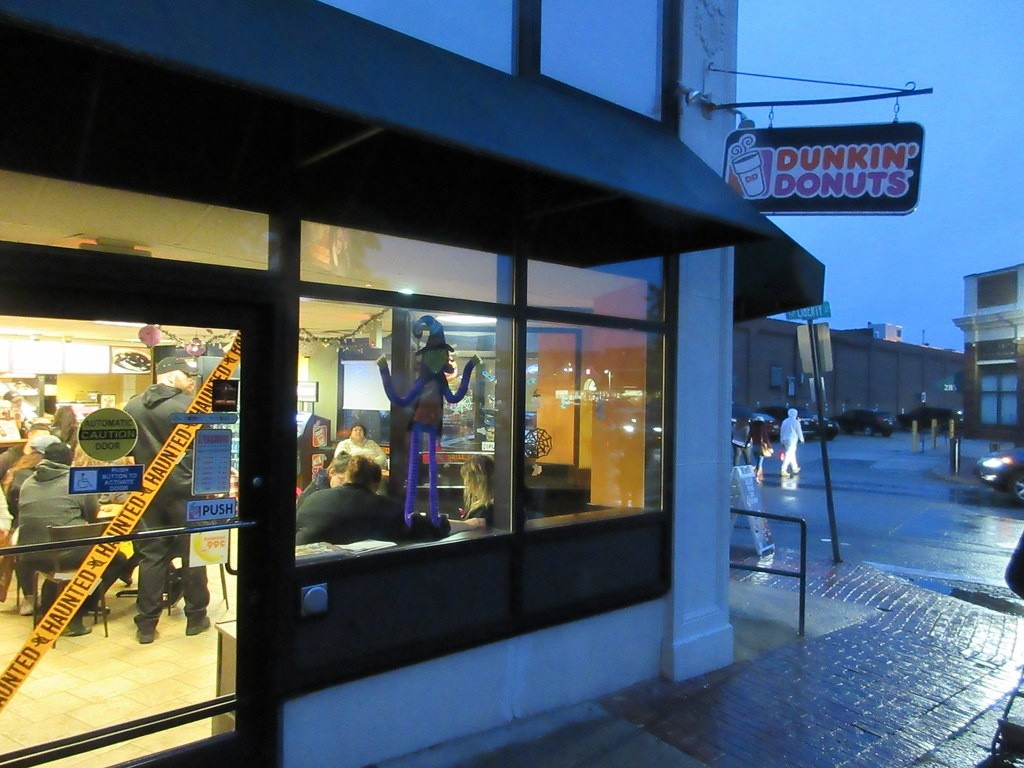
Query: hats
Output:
[[30, 435, 72, 455], [44, 442, 74, 465], [156, 356, 199, 374]]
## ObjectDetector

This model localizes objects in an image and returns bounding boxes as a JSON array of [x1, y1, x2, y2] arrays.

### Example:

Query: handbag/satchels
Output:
[[761, 442, 772, 458], [777, 449, 785, 461]]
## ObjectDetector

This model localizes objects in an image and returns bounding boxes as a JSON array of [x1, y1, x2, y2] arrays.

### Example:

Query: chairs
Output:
[[32, 522, 110, 649]]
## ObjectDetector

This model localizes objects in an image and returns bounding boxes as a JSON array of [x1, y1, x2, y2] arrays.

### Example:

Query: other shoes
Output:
[[793, 467, 801, 474], [66, 625, 92, 636], [780, 472, 790, 476], [20, 595, 38, 615], [186, 617, 210, 635], [137, 622, 155, 643]]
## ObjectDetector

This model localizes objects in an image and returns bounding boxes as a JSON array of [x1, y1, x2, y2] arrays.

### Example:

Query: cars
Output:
[[732, 402, 962, 440], [976, 446, 1024, 505]]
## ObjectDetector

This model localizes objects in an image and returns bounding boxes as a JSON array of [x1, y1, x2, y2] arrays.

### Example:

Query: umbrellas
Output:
[[749, 413, 774, 421]]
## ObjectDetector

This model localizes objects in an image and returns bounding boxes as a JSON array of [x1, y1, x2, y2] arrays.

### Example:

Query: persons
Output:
[[460, 455, 527, 526], [296, 455, 486, 544], [780, 409, 805, 475], [298, 451, 351, 500], [745, 416, 771, 484], [0, 405, 127, 635], [121, 357, 210, 644], [732, 415, 753, 465], [334, 424, 386, 465]]
